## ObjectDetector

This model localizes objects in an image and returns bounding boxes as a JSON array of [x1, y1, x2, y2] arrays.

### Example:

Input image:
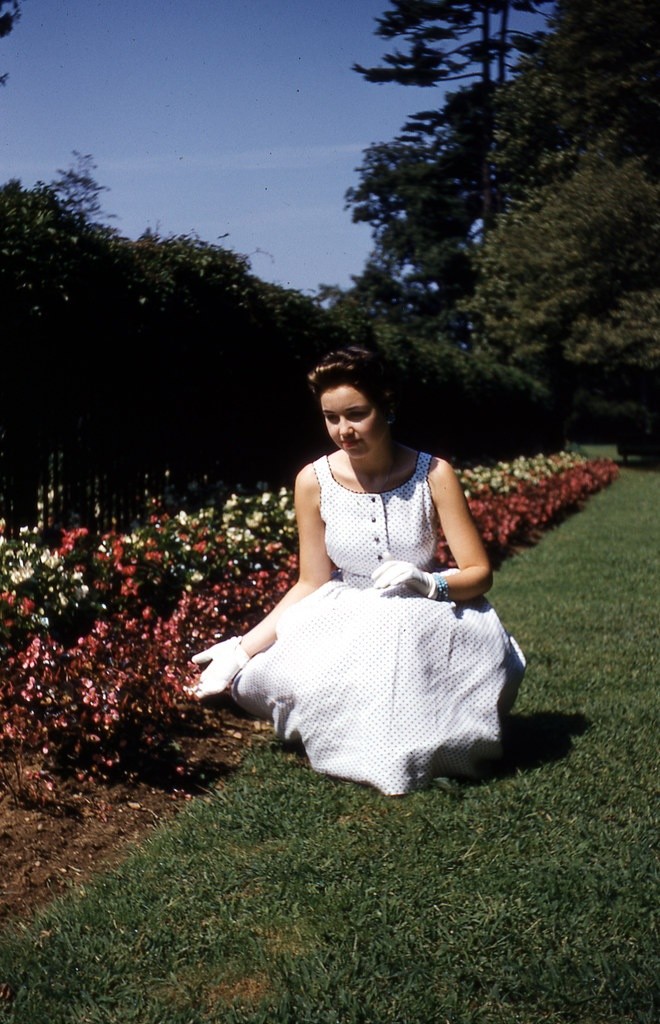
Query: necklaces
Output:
[[378, 457, 394, 491]]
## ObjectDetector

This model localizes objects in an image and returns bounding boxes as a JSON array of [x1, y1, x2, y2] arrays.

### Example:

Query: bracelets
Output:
[[433, 573, 449, 602]]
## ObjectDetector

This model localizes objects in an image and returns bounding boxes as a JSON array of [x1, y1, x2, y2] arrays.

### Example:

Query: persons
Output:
[[183, 354, 527, 797]]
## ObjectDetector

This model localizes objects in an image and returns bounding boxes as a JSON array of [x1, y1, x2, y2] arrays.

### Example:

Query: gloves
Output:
[[371, 560, 438, 599], [183, 636, 251, 698]]
